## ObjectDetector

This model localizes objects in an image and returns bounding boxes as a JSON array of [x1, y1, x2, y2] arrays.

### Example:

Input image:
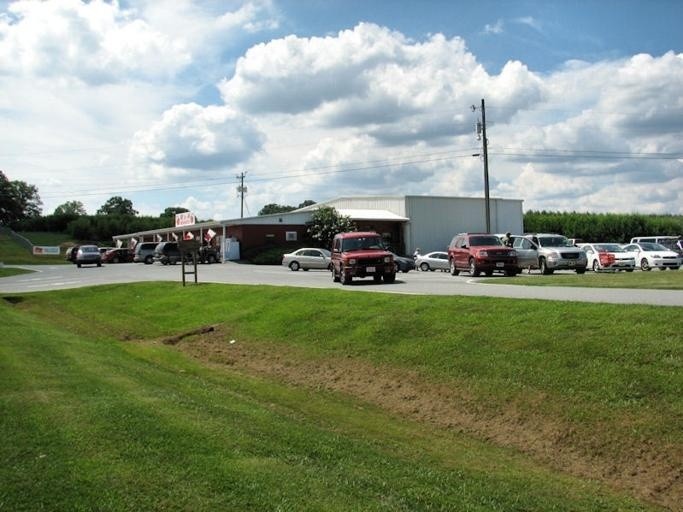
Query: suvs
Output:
[[447, 232, 522, 277], [331, 230, 395, 284]]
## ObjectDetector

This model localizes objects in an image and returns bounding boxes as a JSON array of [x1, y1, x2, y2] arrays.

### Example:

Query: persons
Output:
[[412, 247, 420, 271], [526, 237, 538, 274], [501, 232, 512, 248]]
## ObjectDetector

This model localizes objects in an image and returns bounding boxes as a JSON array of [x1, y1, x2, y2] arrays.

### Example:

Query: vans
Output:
[[630, 235, 683, 248]]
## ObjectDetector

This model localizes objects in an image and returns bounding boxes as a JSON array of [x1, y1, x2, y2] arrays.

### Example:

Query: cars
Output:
[[622, 241, 680, 271], [64, 241, 220, 266], [577, 242, 635, 272], [414, 251, 451, 271], [281, 247, 331, 271], [391, 251, 414, 273], [509, 233, 587, 275]]
